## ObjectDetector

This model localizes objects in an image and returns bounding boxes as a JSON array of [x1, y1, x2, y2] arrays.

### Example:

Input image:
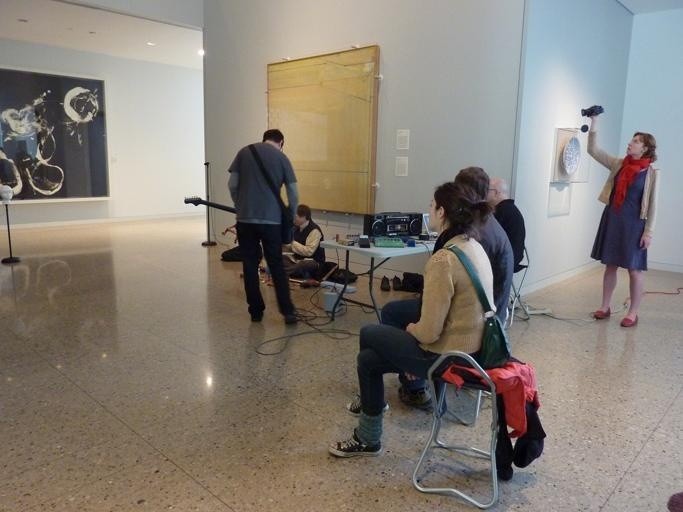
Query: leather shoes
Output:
[[594, 307, 610, 319], [621, 315, 638, 327], [381, 275, 390, 291], [393, 275, 401, 290]]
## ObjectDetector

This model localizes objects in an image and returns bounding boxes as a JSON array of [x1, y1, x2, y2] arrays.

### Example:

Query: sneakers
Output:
[[345, 392, 389, 416], [284, 314, 297, 323], [327, 428, 383, 458], [251, 312, 263, 321], [398, 374, 433, 408]]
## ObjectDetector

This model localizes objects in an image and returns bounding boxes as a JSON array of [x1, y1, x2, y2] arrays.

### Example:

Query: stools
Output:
[[410, 350, 502, 511], [502, 247, 529, 329]]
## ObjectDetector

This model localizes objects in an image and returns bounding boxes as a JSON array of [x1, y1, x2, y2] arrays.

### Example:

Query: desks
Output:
[[319, 237, 435, 326]]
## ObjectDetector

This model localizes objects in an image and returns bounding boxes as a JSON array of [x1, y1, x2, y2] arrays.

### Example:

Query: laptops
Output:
[[423, 214, 437, 235]]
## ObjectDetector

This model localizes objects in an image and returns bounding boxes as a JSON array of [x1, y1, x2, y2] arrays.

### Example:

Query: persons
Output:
[[227, 129, 297, 324], [326, 181, 497, 458], [380, 167, 514, 409], [587, 115, 661, 327], [282, 205, 326, 280], [483, 178, 527, 272]]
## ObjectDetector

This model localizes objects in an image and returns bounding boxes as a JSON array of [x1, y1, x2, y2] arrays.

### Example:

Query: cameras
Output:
[[581, 104, 605, 117]]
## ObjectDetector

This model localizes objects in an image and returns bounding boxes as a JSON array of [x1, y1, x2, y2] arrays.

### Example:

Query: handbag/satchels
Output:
[[478, 315, 512, 369]]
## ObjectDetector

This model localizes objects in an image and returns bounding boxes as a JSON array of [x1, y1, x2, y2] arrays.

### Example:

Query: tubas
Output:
[[185, 197, 292, 244]]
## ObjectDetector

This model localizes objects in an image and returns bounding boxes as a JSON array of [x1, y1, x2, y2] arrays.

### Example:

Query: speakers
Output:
[[364, 214, 385, 237], [409, 213, 422, 234]]
